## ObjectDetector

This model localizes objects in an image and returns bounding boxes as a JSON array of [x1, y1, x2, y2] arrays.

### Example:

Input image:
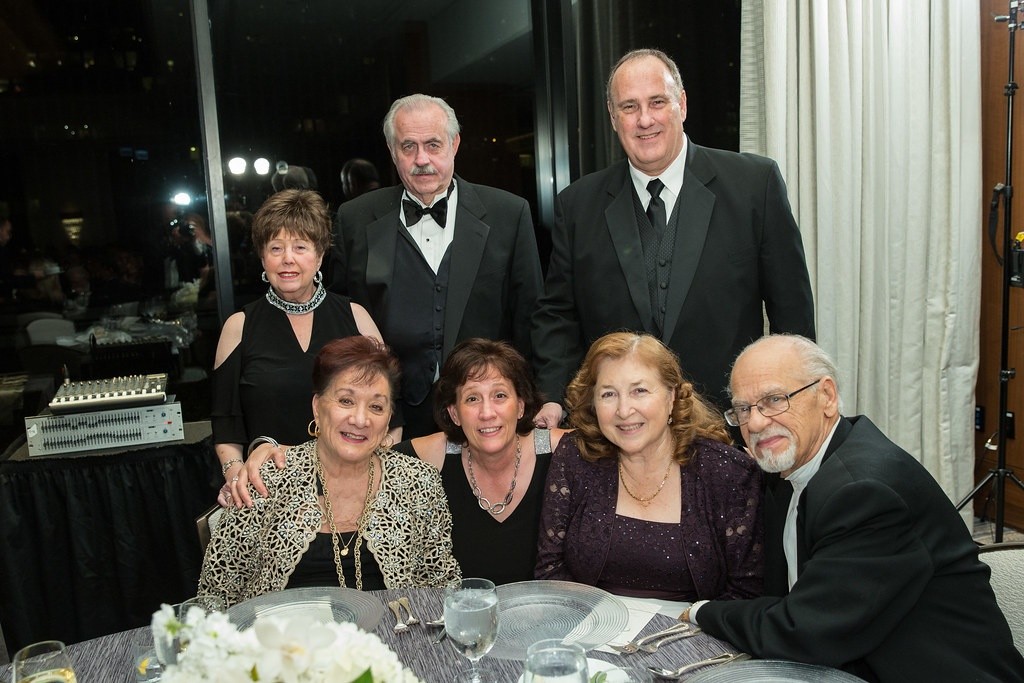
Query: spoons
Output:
[[426, 614, 444, 626], [647, 650, 746, 681]]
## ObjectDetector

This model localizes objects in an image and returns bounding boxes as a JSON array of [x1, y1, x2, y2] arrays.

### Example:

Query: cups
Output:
[[131, 628, 166, 683], [179, 596, 226, 652], [11, 641, 76, 683], [523, 639, 590, 682], [152, 602, 209, 667]]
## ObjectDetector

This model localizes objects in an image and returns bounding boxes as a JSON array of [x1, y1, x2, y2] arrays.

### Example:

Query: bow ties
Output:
[[402, 179, 456, 229]]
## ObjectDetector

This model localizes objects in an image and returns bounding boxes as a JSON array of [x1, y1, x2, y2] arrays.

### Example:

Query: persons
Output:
[[678, 332, 1024, 683], [324, 95, 546, 435], [535, 332, 774, 601], [232, 336, 579, 588], [213, 190, 404, 508], [531, 49, 817, 459], [1, 157, 380, 331], [192, 336, 461, 614]]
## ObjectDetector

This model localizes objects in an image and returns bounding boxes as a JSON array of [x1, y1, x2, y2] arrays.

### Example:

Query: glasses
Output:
[[723, 378, 821, 426]]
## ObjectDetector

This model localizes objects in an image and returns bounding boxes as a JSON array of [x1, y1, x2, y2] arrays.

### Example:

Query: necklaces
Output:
[[617, 437, 675, 506], [315, 446, 379, 590], [466, 435, 523, 514]]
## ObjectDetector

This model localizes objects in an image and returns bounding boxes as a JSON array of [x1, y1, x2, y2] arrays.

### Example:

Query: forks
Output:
[[605, 622, 689, 654], [627, 627, 703, 654], [388, 600, 410, 634], [398, 597, 420, 625]]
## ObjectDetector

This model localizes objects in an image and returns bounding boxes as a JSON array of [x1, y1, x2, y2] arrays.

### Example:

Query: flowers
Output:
[[140, 598, 420, 683]]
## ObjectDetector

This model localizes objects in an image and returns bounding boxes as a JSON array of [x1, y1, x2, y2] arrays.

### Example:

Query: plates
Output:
[[682, 659, 870, 682], [226, 586, 384, 632], [485, 580, 630, 660]]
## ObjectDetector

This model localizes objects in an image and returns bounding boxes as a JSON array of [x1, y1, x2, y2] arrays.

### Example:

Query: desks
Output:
[[0, 420, 224, 660], [0, 589, 762, 683]]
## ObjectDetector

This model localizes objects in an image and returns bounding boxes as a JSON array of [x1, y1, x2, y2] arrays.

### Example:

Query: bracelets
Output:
[[221, 458, 244, 475], [248, 436, 280, 455], [681, 607, 691, 623]]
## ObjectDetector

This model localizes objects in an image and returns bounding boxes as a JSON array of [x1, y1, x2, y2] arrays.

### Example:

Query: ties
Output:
[[645, 178, 667, 240]]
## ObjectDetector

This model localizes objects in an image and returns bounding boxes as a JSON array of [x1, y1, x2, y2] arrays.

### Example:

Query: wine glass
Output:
[[443, 578, 498, 683]]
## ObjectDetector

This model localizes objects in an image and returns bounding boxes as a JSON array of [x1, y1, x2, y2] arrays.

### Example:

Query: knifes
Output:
[[432, 628, 446, 644]]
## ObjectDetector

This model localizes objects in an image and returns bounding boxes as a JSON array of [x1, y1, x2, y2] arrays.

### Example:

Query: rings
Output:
[[225, 497, 231, 500], [232, 475, 240, 481]]
